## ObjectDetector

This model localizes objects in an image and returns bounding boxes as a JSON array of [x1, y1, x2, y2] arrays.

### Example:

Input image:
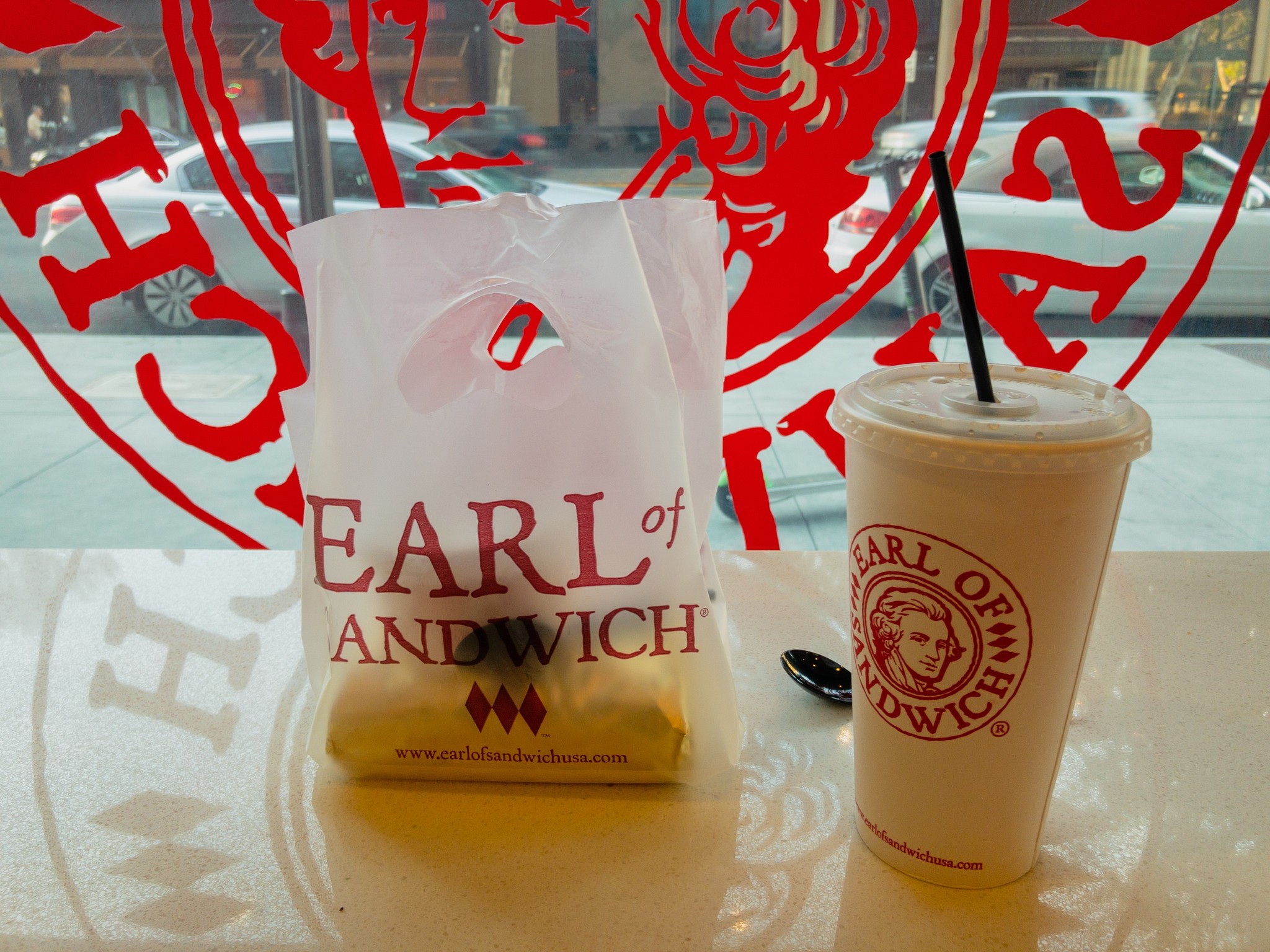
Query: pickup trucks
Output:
[[390, 105, 566, 177]]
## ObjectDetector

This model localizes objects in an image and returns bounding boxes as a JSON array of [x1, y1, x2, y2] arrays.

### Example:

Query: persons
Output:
[[25, 104, 44, 152]]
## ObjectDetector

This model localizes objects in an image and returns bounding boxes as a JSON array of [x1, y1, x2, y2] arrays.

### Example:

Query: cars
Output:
[[39, 121, 647, 335], [828, 88, 1269, 338], [28, 125, 188, 175]]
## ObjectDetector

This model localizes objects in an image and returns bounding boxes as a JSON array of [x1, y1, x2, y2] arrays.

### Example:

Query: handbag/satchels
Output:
[[278, 193, 729, 790]]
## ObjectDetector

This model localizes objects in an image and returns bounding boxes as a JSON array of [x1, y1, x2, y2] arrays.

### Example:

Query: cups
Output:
[[825, 355, 1155, 889]]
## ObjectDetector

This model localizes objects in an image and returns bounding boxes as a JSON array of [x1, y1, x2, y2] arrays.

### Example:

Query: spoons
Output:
[[781, 648, 855, 709]]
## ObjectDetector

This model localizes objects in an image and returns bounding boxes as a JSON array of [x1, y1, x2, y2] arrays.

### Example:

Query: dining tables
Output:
[[3, 549, 1270, 952]]
